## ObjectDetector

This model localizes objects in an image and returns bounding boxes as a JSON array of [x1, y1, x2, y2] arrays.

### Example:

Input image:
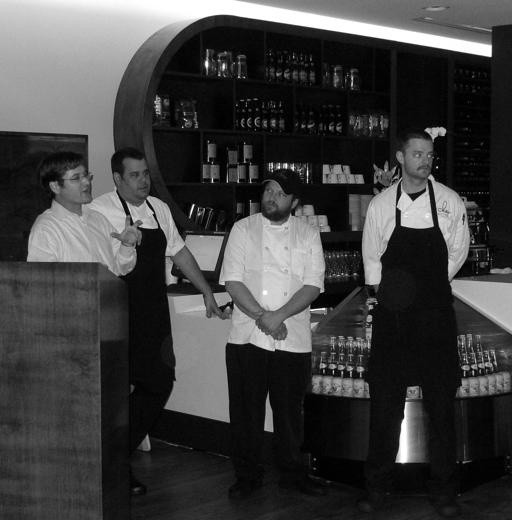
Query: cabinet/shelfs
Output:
[[113, 13, 493, 253]]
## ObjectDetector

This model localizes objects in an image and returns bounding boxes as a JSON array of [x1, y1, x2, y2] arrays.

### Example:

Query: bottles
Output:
[[319, 333, 372, 382], [457, 333, 499, 379], [264, 47, 317, 86], [235, 95, 285, 135], [294, 101, 343, 135]]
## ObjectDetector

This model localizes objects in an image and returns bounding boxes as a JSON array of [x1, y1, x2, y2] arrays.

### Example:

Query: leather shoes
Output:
[[129, 471, 147, 495], [228, 477, 264, 499], [278, 469, 330, 497], [356, 490, 393, 513], [430, 491, 463, 519]]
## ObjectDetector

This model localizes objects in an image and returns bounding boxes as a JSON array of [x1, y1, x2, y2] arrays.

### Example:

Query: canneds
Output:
[[201, 138, 260, 184], [328, 62, 361, 91]]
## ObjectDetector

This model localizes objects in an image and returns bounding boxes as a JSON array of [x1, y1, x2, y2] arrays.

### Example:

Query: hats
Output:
[[261, 169, 304, 196]]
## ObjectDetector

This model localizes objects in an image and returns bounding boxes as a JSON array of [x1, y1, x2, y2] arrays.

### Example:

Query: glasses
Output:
[[60, 172, 94, 182]]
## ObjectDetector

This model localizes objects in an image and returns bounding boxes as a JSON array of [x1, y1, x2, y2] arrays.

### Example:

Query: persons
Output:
[[89, 149, 226, 451], [229, 170, 327, 498], [360, 129, 471, 500], [28, 148, 147, 498]]
